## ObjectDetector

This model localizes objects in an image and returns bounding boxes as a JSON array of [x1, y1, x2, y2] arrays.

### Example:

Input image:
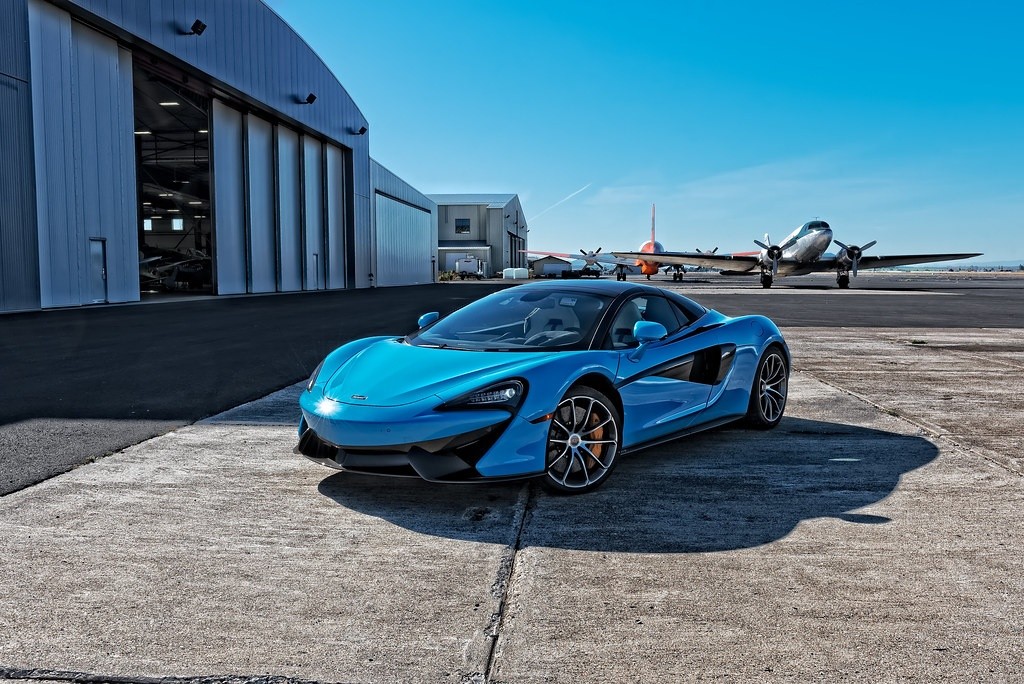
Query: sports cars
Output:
[[294, 278, 793, 498]]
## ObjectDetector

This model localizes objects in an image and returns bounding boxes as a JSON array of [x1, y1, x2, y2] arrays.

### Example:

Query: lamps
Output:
[[189, 18, 208, 37], [300, 93, 317, 105], [357, 126, 367, 136]]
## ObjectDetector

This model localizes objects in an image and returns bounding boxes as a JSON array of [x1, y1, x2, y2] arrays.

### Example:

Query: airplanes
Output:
[[515, 203, 762, 286], [613, 218, 987, 291]]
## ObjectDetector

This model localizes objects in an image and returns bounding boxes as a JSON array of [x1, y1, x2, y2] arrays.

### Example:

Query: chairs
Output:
[[523, 298, 580, 336], [610, 301, 643, 347]]
